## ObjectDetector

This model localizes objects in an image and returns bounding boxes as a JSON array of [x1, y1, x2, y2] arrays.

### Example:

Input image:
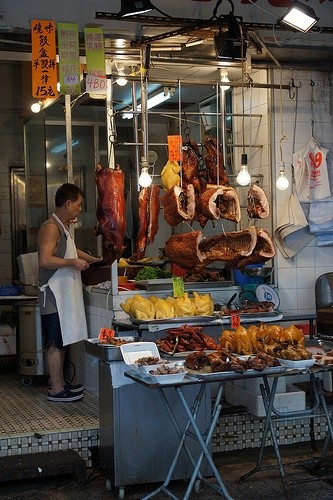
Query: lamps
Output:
[[117, 0, 155, 20], [122, 86, 177, 120], [277, 2, 320, 35], [214, 20, 247, 63]]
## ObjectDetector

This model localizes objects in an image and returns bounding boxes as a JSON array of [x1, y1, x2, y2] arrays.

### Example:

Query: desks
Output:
[[114, 313, 317, 337], [0, 294, 39, 375], [124, 363, 333, 500]]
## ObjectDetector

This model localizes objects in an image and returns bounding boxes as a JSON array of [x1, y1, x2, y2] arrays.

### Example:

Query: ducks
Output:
[[200, 133, 231, 187], [177, 138, 202, 197], [188, 166, 217, 230]]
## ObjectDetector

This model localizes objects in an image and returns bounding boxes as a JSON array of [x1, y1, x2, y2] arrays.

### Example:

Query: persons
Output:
[[38, 183, 101, 402]]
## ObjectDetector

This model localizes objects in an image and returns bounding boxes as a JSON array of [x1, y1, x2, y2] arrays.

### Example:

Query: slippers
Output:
[[47, 388, 84, 401], [48, 382, 84, 392]]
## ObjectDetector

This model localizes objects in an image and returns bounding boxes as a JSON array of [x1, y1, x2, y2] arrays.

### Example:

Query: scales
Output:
[[239, 267, 280, 311]]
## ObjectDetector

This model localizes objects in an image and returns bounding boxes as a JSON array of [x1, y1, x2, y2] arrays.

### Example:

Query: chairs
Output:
[[315, 273, 333, 339]]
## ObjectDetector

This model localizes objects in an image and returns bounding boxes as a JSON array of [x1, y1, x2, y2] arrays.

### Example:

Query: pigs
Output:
[[89, 158, 127, 260]]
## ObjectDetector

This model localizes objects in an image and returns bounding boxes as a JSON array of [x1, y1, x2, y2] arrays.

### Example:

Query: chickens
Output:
[[217, 320, 307, 356]]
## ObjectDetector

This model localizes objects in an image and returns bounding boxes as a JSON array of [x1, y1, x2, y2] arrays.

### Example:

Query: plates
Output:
[[277, 358, 316, 369]]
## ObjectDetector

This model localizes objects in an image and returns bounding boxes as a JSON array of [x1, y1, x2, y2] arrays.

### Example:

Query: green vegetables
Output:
[[135, 265, 172, 280]]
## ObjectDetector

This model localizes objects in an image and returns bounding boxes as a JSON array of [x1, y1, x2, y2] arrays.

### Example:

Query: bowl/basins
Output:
[[149, 371, 188, 383]]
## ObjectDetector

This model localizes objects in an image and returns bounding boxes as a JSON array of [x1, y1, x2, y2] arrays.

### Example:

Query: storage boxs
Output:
[[224, 381, 306, 417], [16, 252, 39, 296]]
